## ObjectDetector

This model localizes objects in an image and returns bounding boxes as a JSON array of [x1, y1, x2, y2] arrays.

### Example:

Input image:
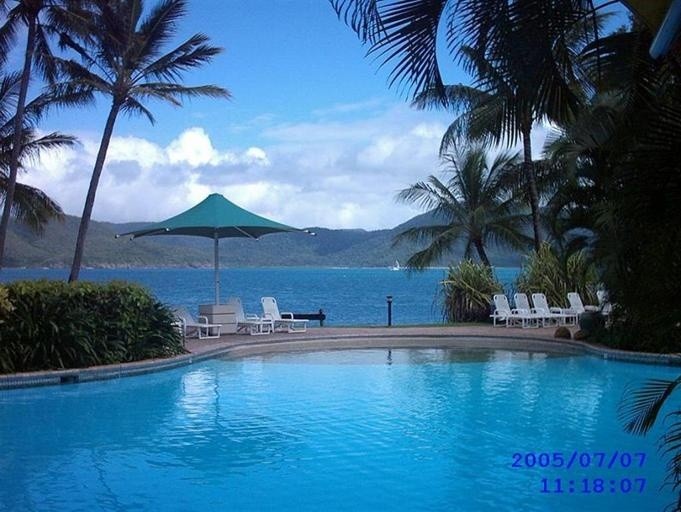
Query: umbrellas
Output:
[[112, 191, 318, 304]]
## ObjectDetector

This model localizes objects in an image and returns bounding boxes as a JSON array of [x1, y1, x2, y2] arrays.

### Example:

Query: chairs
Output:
[[170, 307, 223, 340], [229, 296, 272, 335], [489, 289, 612, 329], [260, 296, 310, 334]]
